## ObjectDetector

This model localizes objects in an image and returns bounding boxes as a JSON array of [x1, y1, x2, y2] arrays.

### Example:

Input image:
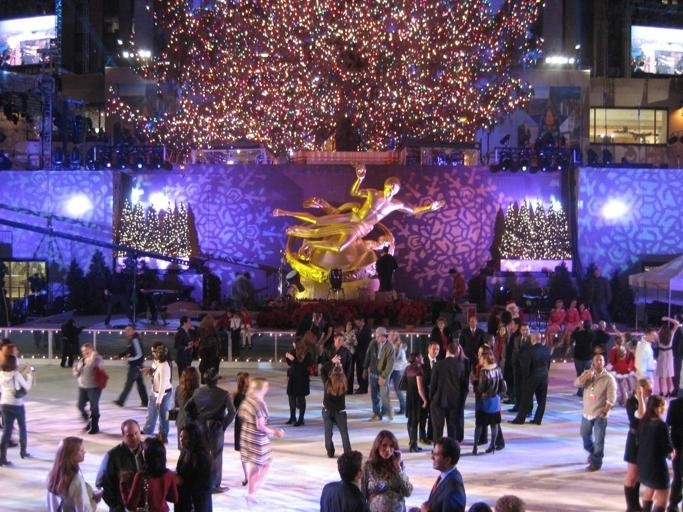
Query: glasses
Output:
[[151, 350, 156, 353]]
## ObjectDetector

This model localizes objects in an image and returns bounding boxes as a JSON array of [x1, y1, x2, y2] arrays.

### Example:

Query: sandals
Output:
[[249, 344, 252, 350], [240, 344, 247, 349]]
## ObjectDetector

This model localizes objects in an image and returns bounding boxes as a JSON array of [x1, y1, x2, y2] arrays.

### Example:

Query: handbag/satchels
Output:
[[498, 368, 508, 394], [123, 475, 150, 512], [92, 355, 109, 390], [169, 385, 181, 422], [12, 374, 28, 400], [398, 367, 408, 392]]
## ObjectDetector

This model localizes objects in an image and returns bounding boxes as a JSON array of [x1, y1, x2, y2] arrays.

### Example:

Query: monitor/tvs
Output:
[[0, 15, 57, 70], [629, 25, 683, 79]]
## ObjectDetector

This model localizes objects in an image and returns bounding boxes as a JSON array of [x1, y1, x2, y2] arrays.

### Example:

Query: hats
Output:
[[205, 368, 223, 383], [375, 325, 390, 338]]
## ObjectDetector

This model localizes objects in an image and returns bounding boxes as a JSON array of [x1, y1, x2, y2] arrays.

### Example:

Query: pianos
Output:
[[140, 289, 177, 294]]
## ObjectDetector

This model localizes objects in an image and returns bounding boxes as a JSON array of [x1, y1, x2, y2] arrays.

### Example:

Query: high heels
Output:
[[242, 479, 248, 484], [244, 495, 255, 502], [473, 447, 476, 455], [485, 442, 496, 453]]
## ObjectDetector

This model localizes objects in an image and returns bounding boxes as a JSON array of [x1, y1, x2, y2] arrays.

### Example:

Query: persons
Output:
[[633, 328, 659, 399], [509, 332, 551, 425], [427, 342, 469, 455], [217, 310, 233, 361], [605, 335, 629, 406], [631, 340, 638, 363], [138, 342, 173, 440], [188, 369, 232, 490], [294, 311, 318, 375], [570, 319, 595, 398], [363, 327, 395, 424], [174, 318, 198, 394], [495, 495, 525, 512], [383, 330, 406, 414], [671, 314, 682, 398], [340, 319, 355, 394], [420, 439, 468, 511], [198, 317, 220, 384], [448, 268, 466, 323], [231, 272, 251, 314], [558, 299, 580, 363], [237, 306, 257, 350], [593, 320, 610, 366], [271, 161, 445, 254], [579, 299, 590, 333], [665, 386, 682, 510], [280, 257, 292, 304], [361, 430, 412, 512], [316, 313, 336, 390], [58, 319, 86, 369], [575, 355, 616, 473], [0, 353, 33, 466], [95, 417, 153, 512], [175, 363, 198, 472], [120, 438, 178, 512], [431, 319, 450, 365], [174, 426, 213, 511], [149, 348, 172, 446], [420, 343, 443, 445], [625, 380, 652, 512], [103, 265, 136, 327], [403, 350, 425, 454], [285, 339, 311, 427], [319, 356, 354, 459], [502, 318, 521, 404], [239, 377, 283, 500], [352, 315, 371, 395], [509, 323, 533, 418], [624, 333, 631, 352], [458, 315, 488, 394], [225, 310, 242, 362], [505, 303, 519, 341], [311, 312, 324, 380], [232, 371, 253, 486], [656, 315, 679, 396], [47, 437, 97, 512], [137, 260, 162, 324], [73, 344, 105, 435], [318, 450, 371, 512], [68, 321, 84, 367], [114, 324, 151, 406], [589, 269, 614, 328], [0, 343, 28, 448], [495, 326, 510, 388], [319, 333, 353, 426], [545, 299, 566, 353], [641, 391, 677, 511], [377, 247, 398, 296]]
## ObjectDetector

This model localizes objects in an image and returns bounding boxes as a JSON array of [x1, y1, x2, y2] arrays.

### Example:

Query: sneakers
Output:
[[367, 414, 382, 423]]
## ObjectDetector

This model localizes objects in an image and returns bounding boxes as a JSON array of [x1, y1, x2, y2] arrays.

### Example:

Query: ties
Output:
[[431, 475, 441, 495], [471, 327, 476, 336], [432, 359, 436, 367], [522, 336, 526, 343]]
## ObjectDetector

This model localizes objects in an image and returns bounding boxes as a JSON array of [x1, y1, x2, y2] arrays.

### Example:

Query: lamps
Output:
[[155, 163, 161, 169], [102, 160, 112, 169], [520, 159, 529, 171], [135, 160, 143, 169], [500, 158, 511, 172]]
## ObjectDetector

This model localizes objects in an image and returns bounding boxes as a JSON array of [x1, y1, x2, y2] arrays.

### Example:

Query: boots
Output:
[[20, 437, 31, 460], [89, 415, 100, 436], [625, 485, 643, 512], [651, 506, 664, 510], [1, 443, 13, 467], [641, 500, 654, 511]]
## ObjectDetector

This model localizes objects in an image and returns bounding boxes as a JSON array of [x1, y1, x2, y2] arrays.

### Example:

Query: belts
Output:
[[658, 346, 674, 351], [646, 368, 657, 372]]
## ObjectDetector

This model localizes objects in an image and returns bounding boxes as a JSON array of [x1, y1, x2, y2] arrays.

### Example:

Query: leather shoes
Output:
[[585, 464, 601, 472], [113, 400, 124, 408], [528, 420, 540, 425], [285, 418, 295, 425], [6, 442, 18, 447], [83, 424, 90, 432], [588, 454, 592, 460], [508, 420, 524, 426], [508, 406, 520, 411], [293, 418, 306, 427], [420, 437, 431, 445], [409, 440, 421, 454]]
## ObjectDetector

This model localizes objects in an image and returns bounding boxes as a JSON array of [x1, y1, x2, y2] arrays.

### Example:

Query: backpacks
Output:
[[92, 366, 107, 389], [123, 492, 155, 512], [15, 384, 29, 400]]
